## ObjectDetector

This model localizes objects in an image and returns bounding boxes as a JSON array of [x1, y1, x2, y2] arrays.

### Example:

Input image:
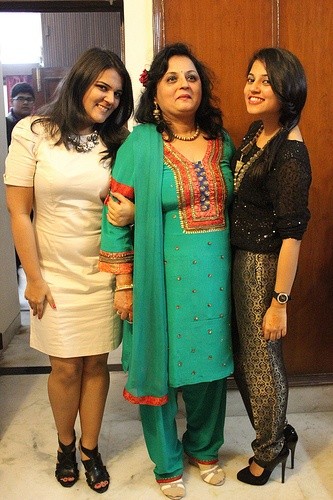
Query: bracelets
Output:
[[115, 284, 133, 292], [129, 224, 135, 230]]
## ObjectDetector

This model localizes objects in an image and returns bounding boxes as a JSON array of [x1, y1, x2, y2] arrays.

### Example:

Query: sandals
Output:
[[78, 438, 110, 493], [159, 478, 186, 499], [55, 428, 79, 488], [189, 459, 226, 486]]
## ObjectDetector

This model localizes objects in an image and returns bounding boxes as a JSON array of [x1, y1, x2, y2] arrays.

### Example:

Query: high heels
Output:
[[237, 444, 289, 486], [248, 424, 298, 469]]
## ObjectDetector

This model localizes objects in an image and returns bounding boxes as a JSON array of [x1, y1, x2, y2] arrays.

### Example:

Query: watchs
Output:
[[272, 290, 292, 304]]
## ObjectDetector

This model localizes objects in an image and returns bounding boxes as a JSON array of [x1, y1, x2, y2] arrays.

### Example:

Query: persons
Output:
[[99, 41, 237, 500], [229, 48, 311, 486], [2, 47, 135, 494]]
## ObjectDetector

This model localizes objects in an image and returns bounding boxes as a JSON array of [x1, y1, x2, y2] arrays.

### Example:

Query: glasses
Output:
[[12, 96, 34, 102]]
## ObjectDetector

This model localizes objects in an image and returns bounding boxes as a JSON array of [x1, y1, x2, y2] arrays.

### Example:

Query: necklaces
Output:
[[164, 120, 200, 141], [67, 129, 99, 153], [234, 125, 284, 195]]
